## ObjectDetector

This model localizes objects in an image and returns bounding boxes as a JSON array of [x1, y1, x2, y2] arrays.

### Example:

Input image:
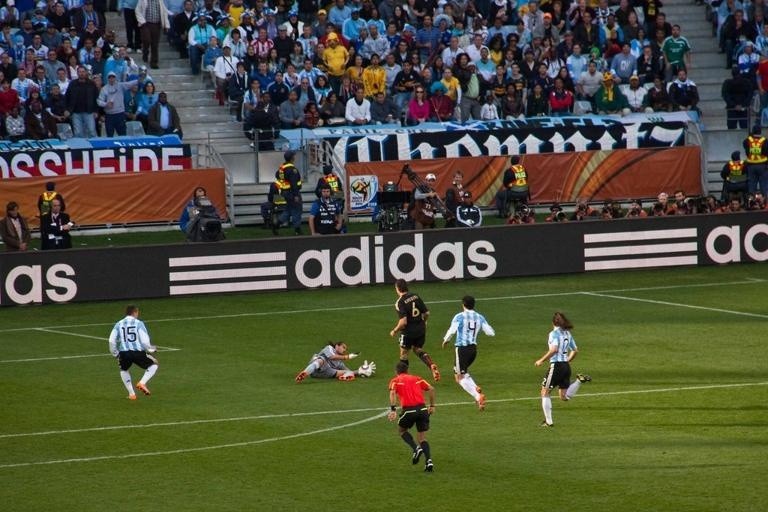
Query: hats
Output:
[[752, 126, 761, 134], [425, 174, 436, 181], [460, 191, 472, 197], [382, 181, 396, 191], [732, 151, 740, 161], [0, 0, 683, 93], [284, 150, 296, 157], [550, 204, 563, 211], [323, 165, 333, 175]]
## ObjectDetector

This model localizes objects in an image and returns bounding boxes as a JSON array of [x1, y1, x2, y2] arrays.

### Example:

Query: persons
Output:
[[720, 125, 768, 213], [180, 187, 215, 243], [37, 182, 75, 250], [168, 1, 703, 151], [260, 151, 720, 253], [0, 1, 182, 144], [442, 295, 495, 412], [108, 305, 159, 400], [388, 361, 435, 472], [390, 278, 440, 381], [1, 202, 31, 251], [295, 341, 377, 383], [534, 312, 591, 427], [707, 0, 768, 131]]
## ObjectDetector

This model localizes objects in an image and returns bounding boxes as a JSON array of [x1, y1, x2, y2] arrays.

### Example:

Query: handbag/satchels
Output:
[[339, 224, 347, 233]]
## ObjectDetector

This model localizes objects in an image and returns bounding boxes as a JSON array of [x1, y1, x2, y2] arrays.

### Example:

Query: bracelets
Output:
[[390, 405, 397, 411]]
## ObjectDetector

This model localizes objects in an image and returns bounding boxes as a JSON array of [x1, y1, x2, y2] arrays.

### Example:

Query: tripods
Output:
[[396, 162, 454, 219]]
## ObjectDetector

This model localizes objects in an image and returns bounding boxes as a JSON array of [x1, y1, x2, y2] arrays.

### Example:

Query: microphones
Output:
[[67, 221, 75, 227], [326, 194, 332, 199]]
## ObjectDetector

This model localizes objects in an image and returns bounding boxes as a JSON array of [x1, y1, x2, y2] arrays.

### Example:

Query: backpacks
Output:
[[40, 191, 58, 216]]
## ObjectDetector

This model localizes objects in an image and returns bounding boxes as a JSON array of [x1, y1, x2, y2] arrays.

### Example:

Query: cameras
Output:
[[631, 208, 640, 215], [677, 200, 687, 208], [655, 203, 663, 212], [226, 71, 232, 77], [579, 204, 586, 211], [750, 200, 757, 207], [554, 211, 566, 222], [602, 206, 612, 214]]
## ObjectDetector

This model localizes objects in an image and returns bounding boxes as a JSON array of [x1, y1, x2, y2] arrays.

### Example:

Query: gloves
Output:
[[348, 351, 361, 359], [364, 360, 376, 374]]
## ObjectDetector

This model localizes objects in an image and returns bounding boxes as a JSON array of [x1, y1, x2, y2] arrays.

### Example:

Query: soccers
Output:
[[358, 365, 371, 379]]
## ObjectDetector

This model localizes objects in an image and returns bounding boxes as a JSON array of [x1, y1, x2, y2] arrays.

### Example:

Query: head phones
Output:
[[318, 183, 333, 195]]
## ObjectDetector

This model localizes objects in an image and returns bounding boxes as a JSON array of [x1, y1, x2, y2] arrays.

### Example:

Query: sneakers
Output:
[[295, 370, 308, 384], [576, 373, 592, 382], [339, 375, 355, 380], [412, 446, 423, 464], [431, 364, 440, 381], [136, 382, 150, 395], [424, 458, 433, 472], [126, 394, 137, 400], [476, 386, 481, 404], [295, 227, 304, 235], [478, 393, 485, 411], [539, 421, 554, 428]]
